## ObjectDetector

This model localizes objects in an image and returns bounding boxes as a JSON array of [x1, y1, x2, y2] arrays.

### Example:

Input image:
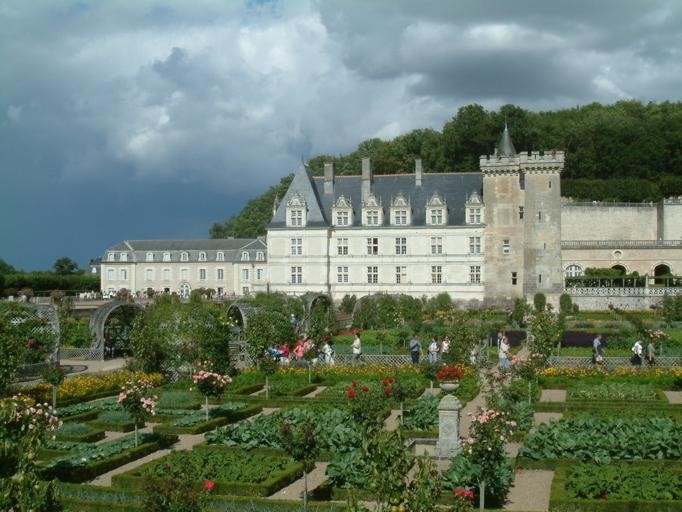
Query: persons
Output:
[[322, 340, 336, 364], [278, 339, 290, 363], [427, 334, 451, 364], [410, 333, 423, 365], [293, 340, 305, 361], [267, 343, 277, 357], [592, 334, 604, 363], [632, 338, 644, 366], [304, 337, 312, 352], [350, 332, 362, 364], [647, 339, 658, 365], [497, 332, 511, 369]]
